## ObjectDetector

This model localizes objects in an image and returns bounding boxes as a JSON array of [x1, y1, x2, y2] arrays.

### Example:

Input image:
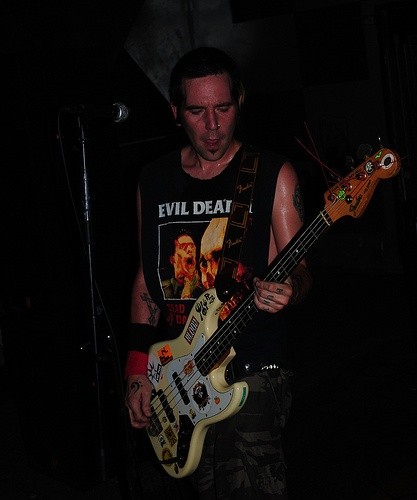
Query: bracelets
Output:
[[127, 323, 157, 377]]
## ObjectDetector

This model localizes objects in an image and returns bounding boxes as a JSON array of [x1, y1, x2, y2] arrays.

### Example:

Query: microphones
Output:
[[61, 102, 130, 123]]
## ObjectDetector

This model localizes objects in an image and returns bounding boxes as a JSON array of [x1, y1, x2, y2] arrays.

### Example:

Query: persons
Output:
[[122, 47, 310, 499]]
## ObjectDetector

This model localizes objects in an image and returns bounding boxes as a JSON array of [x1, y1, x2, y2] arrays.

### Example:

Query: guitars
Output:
[[145, 137, 401, 478]]
